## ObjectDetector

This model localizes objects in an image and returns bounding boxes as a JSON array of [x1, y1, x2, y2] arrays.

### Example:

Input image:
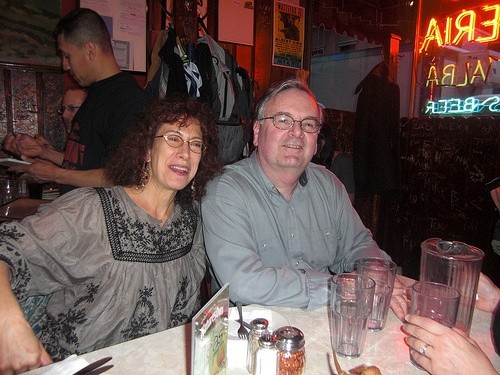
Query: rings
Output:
[[420, 344, 431, 355]]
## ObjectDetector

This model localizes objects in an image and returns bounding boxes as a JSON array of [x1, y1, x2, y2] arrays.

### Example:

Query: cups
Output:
[[330, 273, 376, 360], [420, 237, 484, 338], [356, 256, 397, 331], [3, 179, 15, 200], [410, 281, 461, 330]]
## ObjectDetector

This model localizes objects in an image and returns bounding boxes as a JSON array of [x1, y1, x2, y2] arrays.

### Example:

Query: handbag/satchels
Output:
[[174, 36, 202, 99]]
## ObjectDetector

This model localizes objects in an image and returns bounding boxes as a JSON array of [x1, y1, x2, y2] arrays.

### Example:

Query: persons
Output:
[[2, 8, 144, 194], [0, 93, 221, 375], [201, 80, 411, 324], [403, 271, 500, 375]]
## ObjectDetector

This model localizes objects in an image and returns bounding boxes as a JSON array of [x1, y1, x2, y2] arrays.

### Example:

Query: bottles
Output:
[[273, 326, 307, 375], [246, 318, 272, 374], [252, 334, 281, 375]]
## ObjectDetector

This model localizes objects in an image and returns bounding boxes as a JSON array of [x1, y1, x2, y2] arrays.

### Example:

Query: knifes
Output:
[[74, 356, 111, 375]]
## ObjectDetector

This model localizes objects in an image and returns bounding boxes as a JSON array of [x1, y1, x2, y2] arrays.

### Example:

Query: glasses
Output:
[[154, 134, 208, 154], [259, 114, 323, 133], [57, 104, 80, 114]]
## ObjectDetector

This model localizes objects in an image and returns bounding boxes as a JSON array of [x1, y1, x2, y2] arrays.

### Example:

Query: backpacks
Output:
[[192, 13, 253, 126]]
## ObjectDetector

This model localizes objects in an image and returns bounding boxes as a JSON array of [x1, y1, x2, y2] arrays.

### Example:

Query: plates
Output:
[[228, 306, 290, 337]]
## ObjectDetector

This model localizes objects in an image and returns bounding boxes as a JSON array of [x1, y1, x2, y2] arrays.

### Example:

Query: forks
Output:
[[236, 300, 251, 340]]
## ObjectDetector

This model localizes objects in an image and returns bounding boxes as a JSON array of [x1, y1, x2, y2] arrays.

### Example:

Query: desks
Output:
[[14, 274, 500, 375]]
